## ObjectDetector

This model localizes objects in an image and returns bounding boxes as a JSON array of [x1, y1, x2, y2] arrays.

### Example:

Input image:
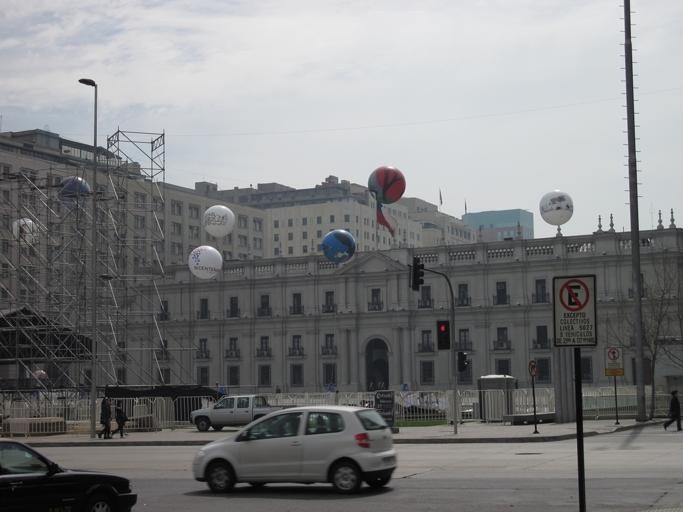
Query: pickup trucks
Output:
[[190, 394, 297, 431]]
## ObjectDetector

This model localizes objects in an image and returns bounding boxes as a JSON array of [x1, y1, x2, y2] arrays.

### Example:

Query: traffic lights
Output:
[[458, 350, 470, 372], [437, 320, 451, 350], [412, 256, 425, 291]]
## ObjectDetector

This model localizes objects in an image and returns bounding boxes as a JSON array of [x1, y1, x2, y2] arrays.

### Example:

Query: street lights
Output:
[[77, 76, 99, 440]]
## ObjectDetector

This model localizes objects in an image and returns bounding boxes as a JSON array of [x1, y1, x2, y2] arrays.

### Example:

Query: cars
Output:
[[191, 405, 399, 494], [0, 437, 139, 512]]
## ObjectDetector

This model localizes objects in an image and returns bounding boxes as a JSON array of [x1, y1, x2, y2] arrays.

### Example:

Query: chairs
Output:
[[239, 400, 248, 408], [366, 390, 397, 422], [271, 416, 338, 436]]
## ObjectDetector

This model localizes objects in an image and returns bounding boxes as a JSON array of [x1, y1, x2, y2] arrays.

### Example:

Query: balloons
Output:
[[186, 245, 223, 282], [57, 177, 91, 208], [321, 229, 356, 265], [30, 370, 50, 389], [9, 218, 40, 250], [540, 190, 574, 227], [364, 164, 407, 205], [203, 204, 236, 239]]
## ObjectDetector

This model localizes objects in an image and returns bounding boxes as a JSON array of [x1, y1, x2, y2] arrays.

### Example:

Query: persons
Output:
[[109, 402, 131, 439], [96, 389, 114, 439], [663, 389, 683, 431]]
